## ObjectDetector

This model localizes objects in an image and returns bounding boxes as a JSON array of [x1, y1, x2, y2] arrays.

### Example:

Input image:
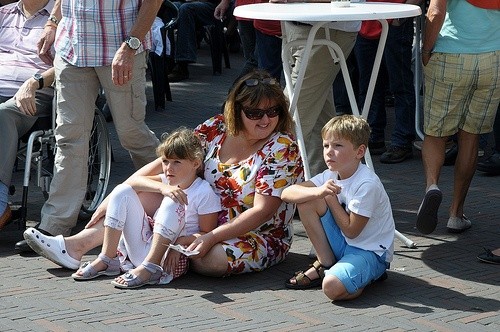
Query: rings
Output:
[[124, 76, 128, 77]]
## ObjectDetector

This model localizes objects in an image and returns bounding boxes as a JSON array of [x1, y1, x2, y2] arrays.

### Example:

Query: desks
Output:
[[233, 2, 422, 249]]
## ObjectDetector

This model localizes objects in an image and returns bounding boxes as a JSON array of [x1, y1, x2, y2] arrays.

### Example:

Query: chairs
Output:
[[141, 1, 178, 113], [203, 9, 235, 78]]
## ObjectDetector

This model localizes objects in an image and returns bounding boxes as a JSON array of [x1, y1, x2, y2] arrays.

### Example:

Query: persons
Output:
[[72, 128, 222, 288], [167, 0, 235, 82], [214, 0, 425, 162], [23, 72, 305, 276], [15, 0, 162, 255], [416, 0, 500, 235], [281, 115, 395, 301], [0, 0, 54, 227]]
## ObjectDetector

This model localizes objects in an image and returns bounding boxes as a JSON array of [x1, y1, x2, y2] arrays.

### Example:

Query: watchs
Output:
[[33, 73, 43, 90], [124, 36, 141, 50]]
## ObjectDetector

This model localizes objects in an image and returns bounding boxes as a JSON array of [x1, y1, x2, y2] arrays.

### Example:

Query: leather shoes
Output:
[[14, 228, 50, 252]]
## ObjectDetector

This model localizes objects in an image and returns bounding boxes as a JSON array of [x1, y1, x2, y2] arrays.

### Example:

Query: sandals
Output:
[[72, 253, 121, 281], [284, 259, 329, 290], [110, 261, 163, 289], [372, 271, 388, 284]]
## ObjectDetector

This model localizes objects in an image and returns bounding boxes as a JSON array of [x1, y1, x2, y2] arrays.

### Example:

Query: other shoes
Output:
[[378, 146, 413, 164], [167, 68, 190, 82], [478, 151, 500, 174], [0, 202, 12, 230], [416, 184, 443, 235], [368, 142, 387, 156], [446, 213, 472, 234], [442, 150, 458, 165], [476, 246, 500, 265]]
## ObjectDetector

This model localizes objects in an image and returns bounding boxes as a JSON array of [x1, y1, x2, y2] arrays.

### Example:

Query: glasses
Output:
[[241, 101, 280, 120], [245, 76, 276, 87]]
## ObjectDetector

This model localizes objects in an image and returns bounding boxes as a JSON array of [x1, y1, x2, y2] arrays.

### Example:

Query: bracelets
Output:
[[46, 17, 59, 28]]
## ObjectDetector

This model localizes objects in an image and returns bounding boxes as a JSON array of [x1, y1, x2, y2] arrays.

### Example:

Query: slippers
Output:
[[25, 227, 80, 269]]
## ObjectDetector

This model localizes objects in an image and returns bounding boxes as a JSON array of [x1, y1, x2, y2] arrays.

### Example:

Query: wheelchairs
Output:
[[0, 89, 112, 231]]
